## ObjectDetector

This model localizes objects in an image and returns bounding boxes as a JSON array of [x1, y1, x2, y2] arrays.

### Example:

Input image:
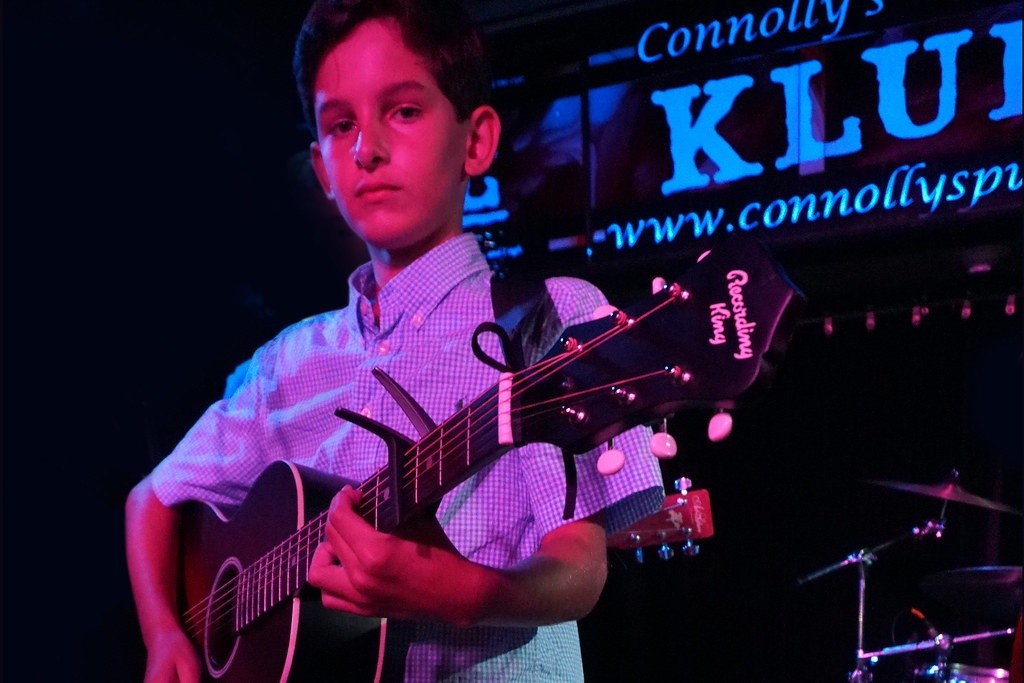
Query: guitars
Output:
[[176, 236, 797, 683], [607, 477, 713, 564]]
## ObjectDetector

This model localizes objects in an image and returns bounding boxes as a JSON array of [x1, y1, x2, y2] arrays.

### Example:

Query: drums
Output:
[[910, 663, 1009, 683]]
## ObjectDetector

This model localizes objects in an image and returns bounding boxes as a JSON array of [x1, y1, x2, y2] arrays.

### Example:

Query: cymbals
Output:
[[862, 479, 1021, 516], [943, 564, 1024, 588]]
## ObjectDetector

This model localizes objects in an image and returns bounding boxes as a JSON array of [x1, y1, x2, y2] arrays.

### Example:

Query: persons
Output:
[[126, 0, 666, 683]]
